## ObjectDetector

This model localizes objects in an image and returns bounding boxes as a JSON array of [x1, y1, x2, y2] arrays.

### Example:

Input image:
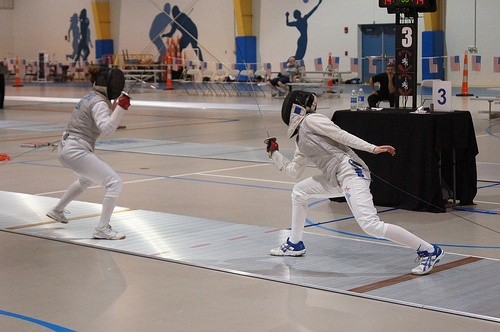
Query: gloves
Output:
[[118, 94, 131, 110], [266, 140, 279, 159]]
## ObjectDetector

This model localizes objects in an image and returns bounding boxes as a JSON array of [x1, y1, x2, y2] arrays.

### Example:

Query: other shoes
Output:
[[274, 91, 281, 98], [283, 91, 289, 98]]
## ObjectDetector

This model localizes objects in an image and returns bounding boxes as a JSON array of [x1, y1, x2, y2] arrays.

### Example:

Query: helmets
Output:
[[281, 90, 317, 138], [92, 67, 126, 100]]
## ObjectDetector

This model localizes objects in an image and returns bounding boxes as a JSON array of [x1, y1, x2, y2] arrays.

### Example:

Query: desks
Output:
[[328, 108, 480, 214]]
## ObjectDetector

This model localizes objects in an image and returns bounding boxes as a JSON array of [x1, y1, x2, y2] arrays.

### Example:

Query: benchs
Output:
[[122, 70, 167, 88], [275, 71, 353, 98]]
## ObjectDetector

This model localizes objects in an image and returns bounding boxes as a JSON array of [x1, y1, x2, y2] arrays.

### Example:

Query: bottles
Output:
[[350, 90, 357, 111], [357, 88, 365, 110]]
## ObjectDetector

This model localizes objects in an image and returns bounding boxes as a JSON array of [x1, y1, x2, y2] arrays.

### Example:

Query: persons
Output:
[[266, 91, 445, 276], [367, 63, 395, 108], [46, 68, 131, 240], [267, 57, 301, 98]]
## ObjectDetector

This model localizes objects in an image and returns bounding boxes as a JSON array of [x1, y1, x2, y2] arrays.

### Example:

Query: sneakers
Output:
[[45, 207, 71, 224], [410, 243, 446, 275], [270, 238, 306, 256], [89, 224, 126, 239]]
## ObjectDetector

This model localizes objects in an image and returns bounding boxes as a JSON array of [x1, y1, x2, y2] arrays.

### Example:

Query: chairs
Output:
[[170, 69, 273, 97]]
